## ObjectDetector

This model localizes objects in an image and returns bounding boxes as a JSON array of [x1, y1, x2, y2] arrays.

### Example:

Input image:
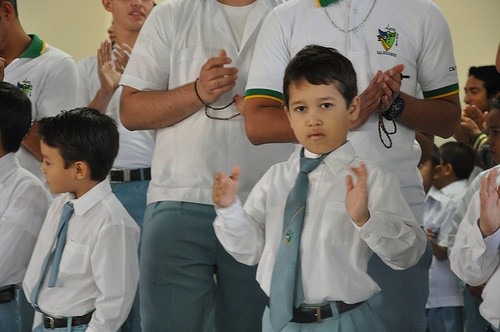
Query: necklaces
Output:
[[324, 0, 376, 33]]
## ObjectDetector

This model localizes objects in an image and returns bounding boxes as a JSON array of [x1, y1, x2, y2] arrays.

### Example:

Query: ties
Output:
[[269, 146, 326, 332], [31, 202, 74, 304]]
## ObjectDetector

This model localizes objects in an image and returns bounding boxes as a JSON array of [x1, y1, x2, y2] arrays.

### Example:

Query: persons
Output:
[[0, 81, 54, 332], [241, 0, 462, 332], [77, 0, 165, 225], [119, 0, 268, 332], [212, 45, 428, 332], [419, 65, 500, 332], [0, 0, 85, 197], [21, 108, 141, 332]]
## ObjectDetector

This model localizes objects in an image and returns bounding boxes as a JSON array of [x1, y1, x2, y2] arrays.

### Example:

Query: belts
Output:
[[266, 296, 368, 325], [110, 167, 152, 183], [0, 287, 16, 304], [44, 308, 95, 330]]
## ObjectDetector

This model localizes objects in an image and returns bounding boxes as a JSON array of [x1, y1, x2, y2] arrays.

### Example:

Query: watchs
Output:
[[383, 97, 405, 120]]
